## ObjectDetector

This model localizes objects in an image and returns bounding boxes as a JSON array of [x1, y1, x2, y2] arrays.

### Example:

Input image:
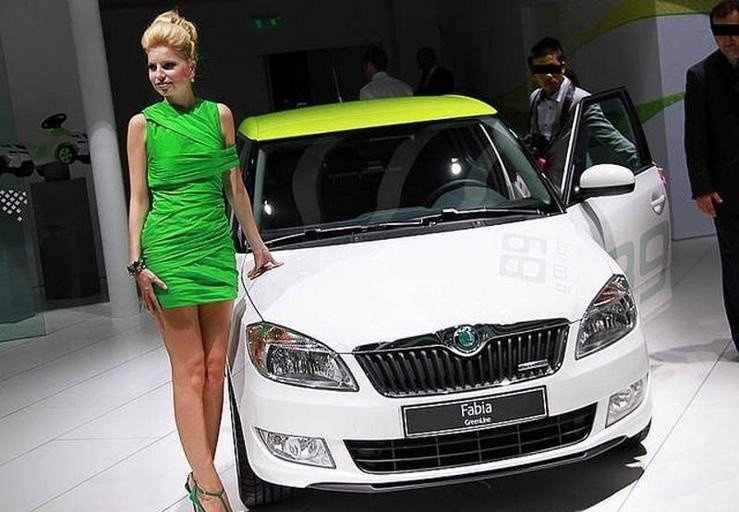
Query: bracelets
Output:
[[126, 258, 149, 276]]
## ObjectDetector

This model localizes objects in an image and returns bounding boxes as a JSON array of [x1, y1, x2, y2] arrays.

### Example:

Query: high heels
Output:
[[184, 471, 233, 512]]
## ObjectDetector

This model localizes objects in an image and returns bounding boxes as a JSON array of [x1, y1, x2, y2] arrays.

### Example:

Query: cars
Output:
[[0, 111, 94, 178], [218, 86, 680, 512]]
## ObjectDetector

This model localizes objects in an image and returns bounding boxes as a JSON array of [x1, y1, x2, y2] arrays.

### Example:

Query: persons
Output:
[[528, 37, 666, 189], [684, 1, 738, 350], [125, 10, 284, 512], [359, 45, 414, 99], [416, 47, 454, 95]]
